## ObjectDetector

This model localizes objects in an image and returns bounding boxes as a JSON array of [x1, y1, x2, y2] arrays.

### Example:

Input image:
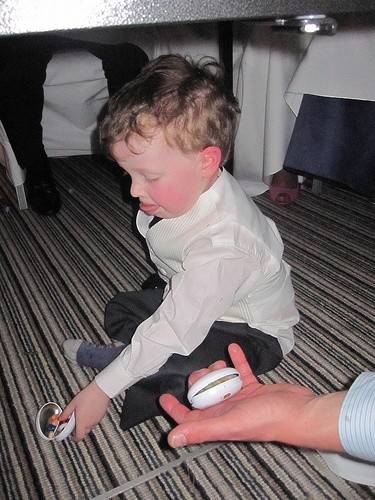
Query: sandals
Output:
[[268, 171, 301, 204]]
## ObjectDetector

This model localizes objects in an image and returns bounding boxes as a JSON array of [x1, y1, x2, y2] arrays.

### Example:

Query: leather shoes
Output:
[[110, 161, 138, 204], [25, 162, 63, 217]]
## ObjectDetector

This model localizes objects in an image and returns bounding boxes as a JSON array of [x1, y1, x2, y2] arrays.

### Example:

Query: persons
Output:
[[0, 35, 151, 215], [58, 53, 300, 442], [270, 170, 299, 205], [158, 342, 375, 462]]
